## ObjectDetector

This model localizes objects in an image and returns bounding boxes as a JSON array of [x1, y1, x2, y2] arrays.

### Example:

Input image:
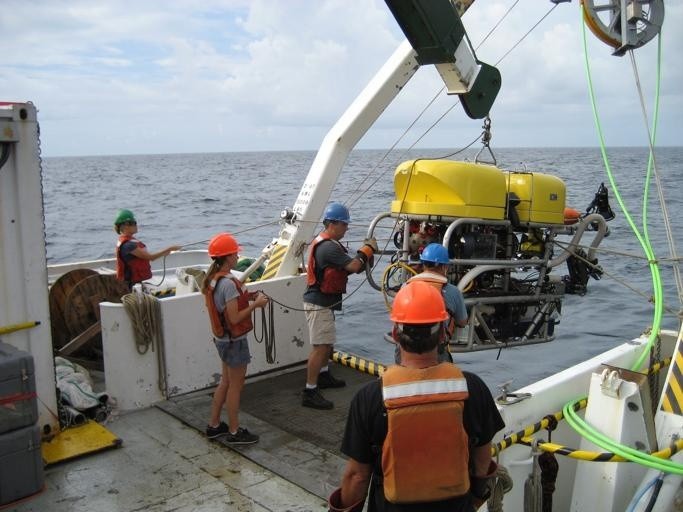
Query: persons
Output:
[[202, 233, 268, 446], [301, 204, 377, 409], [395, 242, 469, 367], [113, 209, 184, 295], [340, 281, 505, 512]]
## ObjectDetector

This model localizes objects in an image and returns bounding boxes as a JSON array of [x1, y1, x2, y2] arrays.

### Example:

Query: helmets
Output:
[[114, 210, 136, 224], [208, 232, 240, 256], [420, 242, 453, 265], [324, 203, 353, 223], [389, 281, 449, 324]]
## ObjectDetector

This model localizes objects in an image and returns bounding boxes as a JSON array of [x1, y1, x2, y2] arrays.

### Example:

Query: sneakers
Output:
[[318, 371, 345, 389], [206, 422, 229, 438], [302, 387, 333, 409], [227, 427, 259, 444]]
